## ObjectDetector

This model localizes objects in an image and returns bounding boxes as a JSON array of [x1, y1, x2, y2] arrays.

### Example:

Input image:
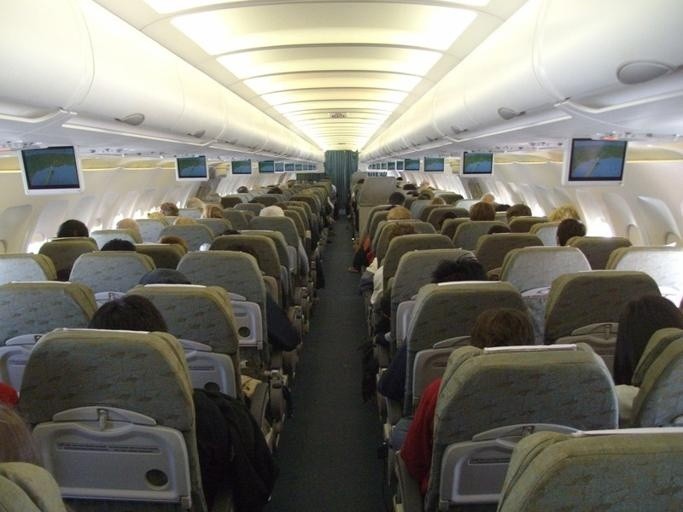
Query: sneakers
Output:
[[349, 266, 359, 273]]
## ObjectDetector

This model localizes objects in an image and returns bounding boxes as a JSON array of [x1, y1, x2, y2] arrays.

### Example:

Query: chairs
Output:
[[1, 174, 341, 510], [347, 170, 683, 512]]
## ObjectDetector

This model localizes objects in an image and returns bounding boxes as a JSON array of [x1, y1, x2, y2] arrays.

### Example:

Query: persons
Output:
[[59, 187, 310, 382], [613, 294, 683, 428], [548, 204, 587, 246], [345, 176, 532, 308], [359, 253, 536, 507], [279, 180, 340, 243], [1, 295, 277, 512]]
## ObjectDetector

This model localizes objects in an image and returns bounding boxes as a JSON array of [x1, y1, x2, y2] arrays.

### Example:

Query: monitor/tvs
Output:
[[460, 151, 495, 177], [229, 159, 319, 175], [560, 136, 628, 187], [367, 157, 446, 172], [16, 144, 85, 197], [175, 155, 210, 181]]
